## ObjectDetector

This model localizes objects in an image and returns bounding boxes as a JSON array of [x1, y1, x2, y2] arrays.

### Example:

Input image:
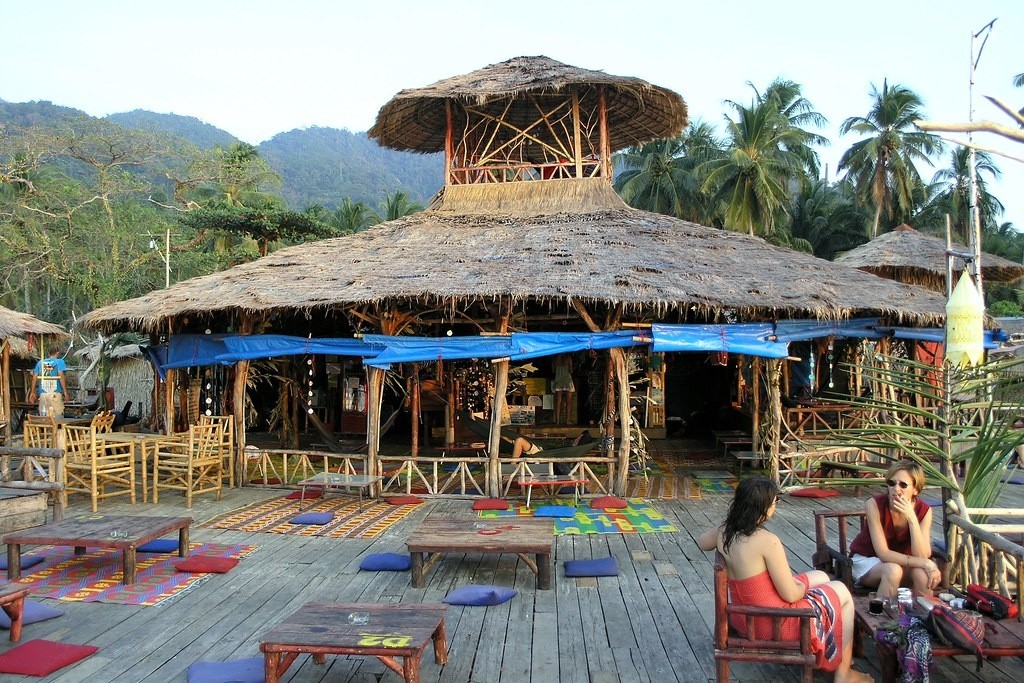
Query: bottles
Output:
[[41, 403, 47, 416]]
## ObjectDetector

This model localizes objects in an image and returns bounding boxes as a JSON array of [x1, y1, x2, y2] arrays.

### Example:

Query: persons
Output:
[[848, 459, 942, 599], [509, 429, 593, 465], [551, 352, 578, 424], [700, 476, 875, 683], [28, 343, 69, 419]]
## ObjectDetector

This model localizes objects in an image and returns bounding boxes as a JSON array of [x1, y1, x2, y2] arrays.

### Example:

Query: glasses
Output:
[[887, 480, 915, 489], [771, 496, 781, 506]]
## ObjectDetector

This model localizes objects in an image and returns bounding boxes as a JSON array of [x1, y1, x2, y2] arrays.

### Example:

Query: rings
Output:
[[933, 580, 937, 582]]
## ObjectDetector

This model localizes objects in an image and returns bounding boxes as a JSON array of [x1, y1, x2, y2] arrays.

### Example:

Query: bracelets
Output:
[[906, 555, 911, 568], [926, 562, 938, 573]]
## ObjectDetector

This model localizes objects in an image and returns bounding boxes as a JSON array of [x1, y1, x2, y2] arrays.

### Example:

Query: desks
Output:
[[730, 451, 770, 479], [298, 472, 384, 513], [335, 439, 365, 445], [902, 452, 967, 479], [854, 592, 1023, 683], [502, 464, 554, 476], [79, 432, 188, 503], [435, 444, 488, 466], [257, 517, 555, 683], [723, 438, 762, 461], [517, 476, 590, 510], [821, 461, 893, 496], [714, 431, 751, 449], [32, 417, 93, 440], [3, 512, 193, 585], [527, 434, 566, 447], [310, 441, 354, 451]]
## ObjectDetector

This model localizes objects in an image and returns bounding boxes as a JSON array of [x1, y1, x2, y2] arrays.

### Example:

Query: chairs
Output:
[[24, 408, 229, 508], [712, 510, 952, 683], [113, 401, 143, 428]]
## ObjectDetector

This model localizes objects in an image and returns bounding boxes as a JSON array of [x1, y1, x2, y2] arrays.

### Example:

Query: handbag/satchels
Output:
[[962, 584, 1019, 621], [924, 604, 998, 672]]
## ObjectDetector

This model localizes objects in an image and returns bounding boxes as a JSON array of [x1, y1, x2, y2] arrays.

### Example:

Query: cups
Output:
[[869, 591, 883, 616]]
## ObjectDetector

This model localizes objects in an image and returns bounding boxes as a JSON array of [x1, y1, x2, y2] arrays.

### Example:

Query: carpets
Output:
[[0, 433, 799, 607]]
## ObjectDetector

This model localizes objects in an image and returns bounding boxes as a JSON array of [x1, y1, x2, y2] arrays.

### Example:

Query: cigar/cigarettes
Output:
[[898, 492, 902, 496]]
